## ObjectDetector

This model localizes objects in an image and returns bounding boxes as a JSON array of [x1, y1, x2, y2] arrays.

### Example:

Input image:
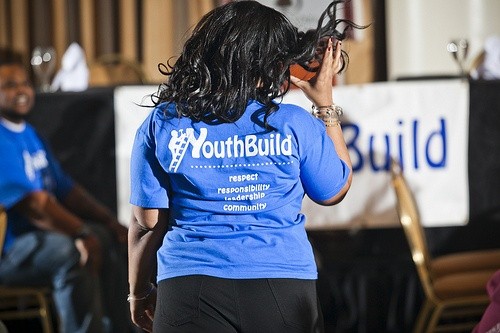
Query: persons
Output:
[[0, 49, 128, 333], [128, 0, 373, 333]]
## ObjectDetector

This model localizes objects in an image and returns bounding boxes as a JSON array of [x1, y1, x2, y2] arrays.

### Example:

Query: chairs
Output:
[[390, 160, 500, 333], [0, 288, 54, 333]]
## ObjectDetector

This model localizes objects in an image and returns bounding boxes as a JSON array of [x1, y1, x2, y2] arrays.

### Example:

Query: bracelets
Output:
[[310, 103, 345, 127], [126, 282, 154, 302]]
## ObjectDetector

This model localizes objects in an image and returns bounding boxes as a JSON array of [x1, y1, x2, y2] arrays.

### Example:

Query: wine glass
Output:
[[449, 36, 470, 79], [31, 47, 56, 94]]
